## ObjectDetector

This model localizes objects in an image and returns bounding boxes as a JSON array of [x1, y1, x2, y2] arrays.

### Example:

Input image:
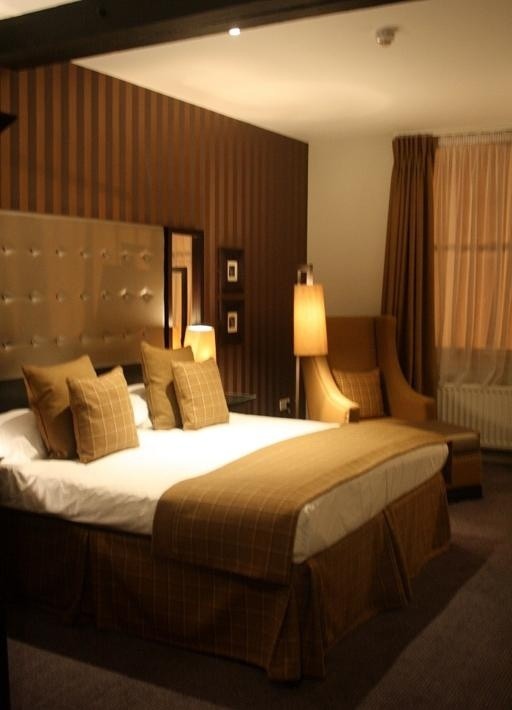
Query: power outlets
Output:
[[279, 398, 290, 412]]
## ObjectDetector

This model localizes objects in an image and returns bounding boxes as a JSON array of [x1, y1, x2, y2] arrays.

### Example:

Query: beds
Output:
[[3, 363, 455, 684]]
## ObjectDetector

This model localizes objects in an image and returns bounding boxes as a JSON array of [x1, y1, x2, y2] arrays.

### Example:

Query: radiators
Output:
[[442, 381, 511, 451]]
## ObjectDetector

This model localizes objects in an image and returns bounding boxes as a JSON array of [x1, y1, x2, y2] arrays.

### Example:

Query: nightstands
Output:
[[220, 393, 257, 417]]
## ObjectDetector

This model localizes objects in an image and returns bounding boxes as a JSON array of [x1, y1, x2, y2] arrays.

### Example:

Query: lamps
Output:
[[184, 323, 217, 363], [291, 264, 330, 420]]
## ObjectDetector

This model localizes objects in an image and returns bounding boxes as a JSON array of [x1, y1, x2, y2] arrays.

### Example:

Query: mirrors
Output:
[[171, 266, 188, 351], [159, 225, 205, 354]]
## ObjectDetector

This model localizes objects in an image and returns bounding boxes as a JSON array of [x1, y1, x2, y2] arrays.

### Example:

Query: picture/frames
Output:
[[218, 247, 246, 294], [217, 298, 246, 347]]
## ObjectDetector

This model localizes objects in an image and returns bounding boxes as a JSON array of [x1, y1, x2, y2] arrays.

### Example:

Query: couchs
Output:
[[307, 315, 436, 427], [360, 418, 487, 497]]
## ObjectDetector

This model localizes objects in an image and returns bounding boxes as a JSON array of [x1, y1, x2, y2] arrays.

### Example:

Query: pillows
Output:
[[65, 366, 142, 463], [20, 354, 103, 460], [140, 342, 200, 431], [172, 354, 234, 429], [330, 367, 387, 422]]
